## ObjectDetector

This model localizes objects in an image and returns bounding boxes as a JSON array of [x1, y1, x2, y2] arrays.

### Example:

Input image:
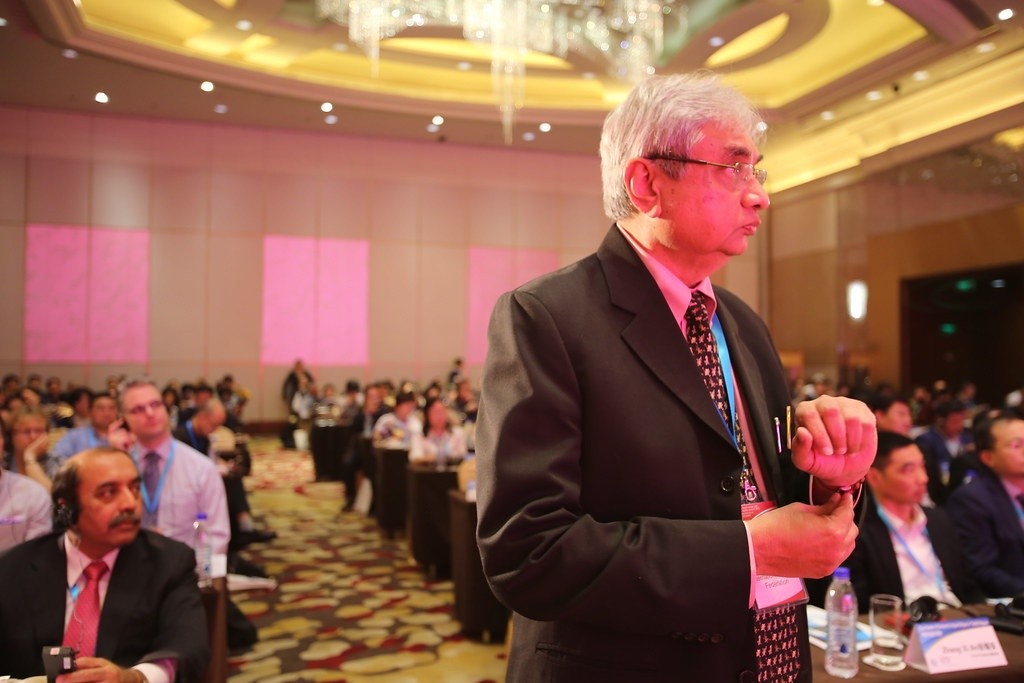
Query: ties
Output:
[[682, 291, 803, 683], [138, 452, 162, 527], [58, 561, 109, 683]]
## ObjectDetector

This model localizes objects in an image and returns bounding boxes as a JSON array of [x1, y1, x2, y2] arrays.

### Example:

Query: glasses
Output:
[[642, 151, 766, 187]]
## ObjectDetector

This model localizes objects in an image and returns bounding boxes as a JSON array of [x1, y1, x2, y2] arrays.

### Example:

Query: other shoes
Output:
[[241, 525, 277, 546]]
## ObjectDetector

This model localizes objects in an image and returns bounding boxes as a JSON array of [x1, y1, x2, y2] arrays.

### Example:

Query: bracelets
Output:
[[817, 477, 865, 494]]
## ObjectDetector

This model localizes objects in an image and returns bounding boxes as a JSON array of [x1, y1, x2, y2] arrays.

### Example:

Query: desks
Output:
[[308, 419, 345, 482], [373, 441, 410, 543], [450, 490, 509, 646], [408, 461, 459, 584], [809, 603, 1024, 683]]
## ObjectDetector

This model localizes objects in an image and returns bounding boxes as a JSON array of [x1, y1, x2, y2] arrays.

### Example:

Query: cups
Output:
[[870, 595, 903, 665]]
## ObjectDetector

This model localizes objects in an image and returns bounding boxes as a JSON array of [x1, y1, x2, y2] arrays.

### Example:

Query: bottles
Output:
[[824, 568, 858, 679], [193, 513, 211, 587]]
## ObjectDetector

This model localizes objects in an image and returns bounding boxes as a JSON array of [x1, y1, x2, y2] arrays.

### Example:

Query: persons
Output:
[[474, 74, 877, 683], [1, 352, 1022, 653], [1, 447, 207, 683]]
